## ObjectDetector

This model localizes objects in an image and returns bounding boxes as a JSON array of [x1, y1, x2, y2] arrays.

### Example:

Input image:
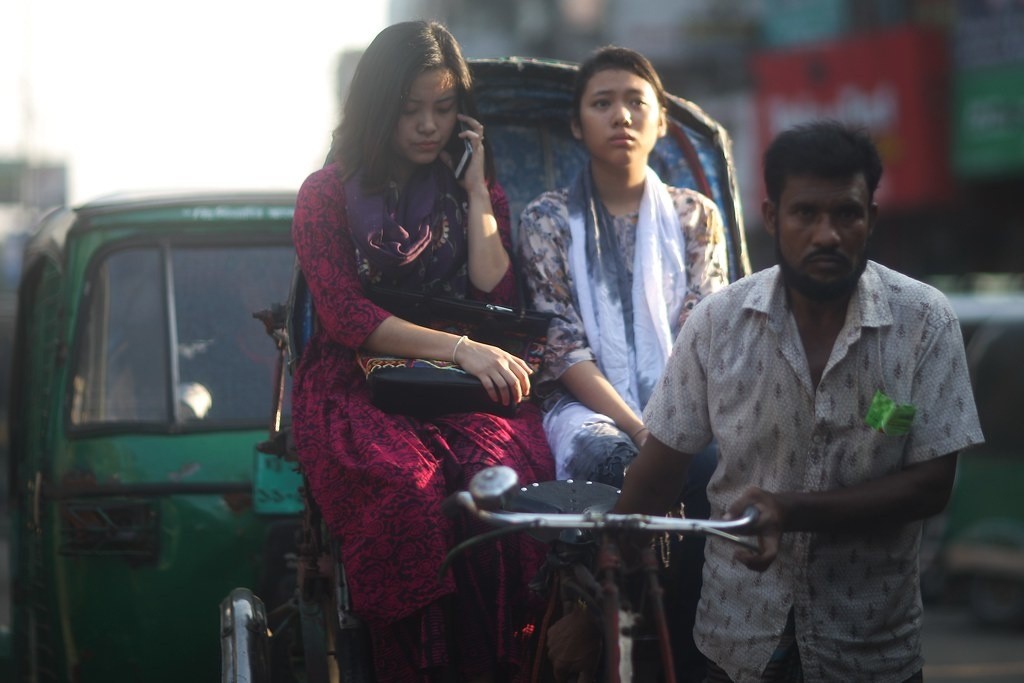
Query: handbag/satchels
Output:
[[352, 244, 573, 419]]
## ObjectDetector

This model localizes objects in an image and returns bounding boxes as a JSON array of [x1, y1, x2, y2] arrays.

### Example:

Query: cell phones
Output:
[[446, 120, 473, 181]]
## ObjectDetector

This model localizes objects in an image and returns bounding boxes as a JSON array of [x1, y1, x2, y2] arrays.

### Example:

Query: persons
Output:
[[547, 122, 986, 683], [516, 47, 732, 683], [292, 22, 558, 683]]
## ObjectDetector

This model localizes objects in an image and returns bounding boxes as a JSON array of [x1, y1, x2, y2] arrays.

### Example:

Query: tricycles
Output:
[[220, 55, 763, 683], [0, 190, 329, 683]]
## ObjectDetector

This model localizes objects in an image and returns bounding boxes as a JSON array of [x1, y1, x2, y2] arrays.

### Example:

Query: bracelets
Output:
[[451, 334, 469, 364], [578, 595, 601, 624], [630, 427, 647, 439]]
